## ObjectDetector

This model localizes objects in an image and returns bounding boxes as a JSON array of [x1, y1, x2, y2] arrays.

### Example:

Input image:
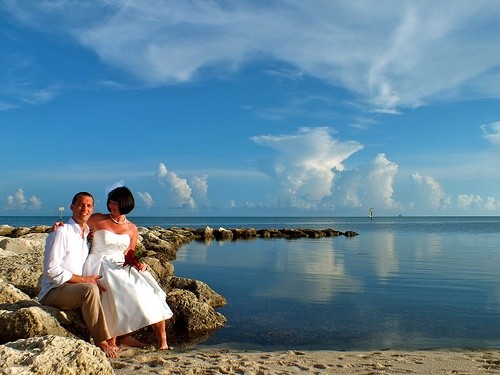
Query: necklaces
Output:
[[109, 213, 127, 224]]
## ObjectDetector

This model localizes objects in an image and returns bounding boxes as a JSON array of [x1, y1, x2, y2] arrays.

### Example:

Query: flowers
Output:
[[115, 244, 150, 278]]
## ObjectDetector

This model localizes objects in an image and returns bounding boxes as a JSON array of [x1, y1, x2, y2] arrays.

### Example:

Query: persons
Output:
[[49, 186, 170, 353], [34, 192, 146, 358]]
